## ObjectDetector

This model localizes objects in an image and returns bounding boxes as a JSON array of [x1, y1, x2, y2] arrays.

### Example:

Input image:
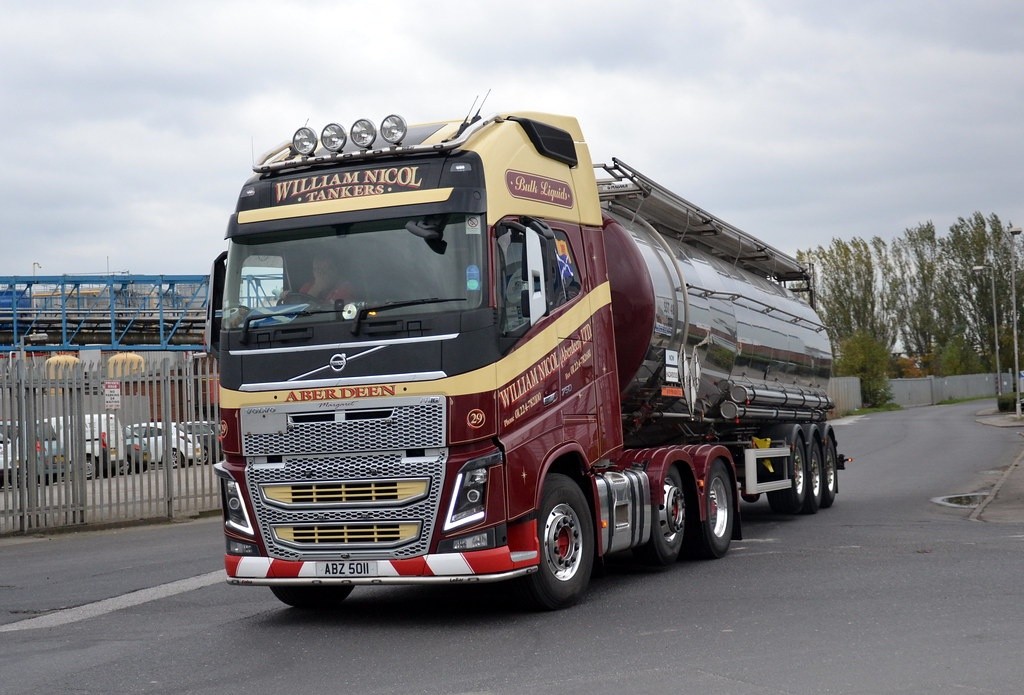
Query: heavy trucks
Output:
[[212, 107, 847, 611]]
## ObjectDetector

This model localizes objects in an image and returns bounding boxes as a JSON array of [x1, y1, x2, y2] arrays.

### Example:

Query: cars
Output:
[[125, 421, 202, 472], [0, 419, 64, 485], [45, 414, 125, 482], [179, 421, 226, 465]]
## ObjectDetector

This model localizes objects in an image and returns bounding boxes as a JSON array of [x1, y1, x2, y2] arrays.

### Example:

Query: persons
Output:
[[280, 248, 356, 305]]
